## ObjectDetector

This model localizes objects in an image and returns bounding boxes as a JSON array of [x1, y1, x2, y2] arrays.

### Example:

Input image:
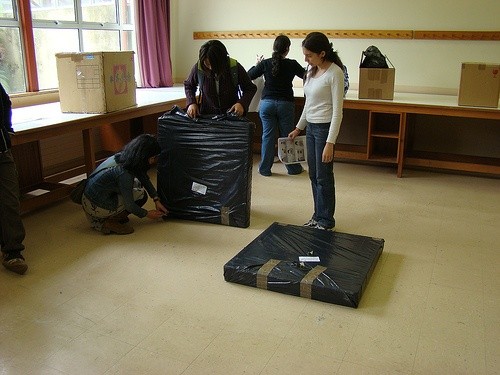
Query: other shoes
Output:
[[3, 255, 28, 274]]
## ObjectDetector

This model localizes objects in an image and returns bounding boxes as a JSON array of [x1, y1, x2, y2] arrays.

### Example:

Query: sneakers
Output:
[[303, 218, 336, 231]]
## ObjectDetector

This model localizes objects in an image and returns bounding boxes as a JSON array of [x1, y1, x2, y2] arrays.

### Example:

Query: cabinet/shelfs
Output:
[[11, 82, 500, 217]]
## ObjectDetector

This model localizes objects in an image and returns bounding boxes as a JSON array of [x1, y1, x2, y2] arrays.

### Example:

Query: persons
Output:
[[288, 32, 345, 231], [81, 134, 168, 235], [247, 35, 308, 176], [183, 40, 258, 118], [0, 82, 28, 273]]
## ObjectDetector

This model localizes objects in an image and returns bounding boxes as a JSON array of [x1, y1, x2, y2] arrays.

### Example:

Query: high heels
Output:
[[102, 220, 134, 234]]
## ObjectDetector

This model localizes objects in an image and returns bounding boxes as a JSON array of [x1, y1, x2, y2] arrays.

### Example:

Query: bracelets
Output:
[[153, 198, 160, 202]]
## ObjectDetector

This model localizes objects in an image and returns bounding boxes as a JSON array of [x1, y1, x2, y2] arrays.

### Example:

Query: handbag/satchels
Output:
[[69, 179, 89, 205], [360, 45, 395, 68]]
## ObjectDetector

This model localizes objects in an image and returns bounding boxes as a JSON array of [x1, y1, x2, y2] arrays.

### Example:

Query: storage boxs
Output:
[[458, 62, 500, 108], [55, 51, 136, 114], [358, 66, 395, 100]]
